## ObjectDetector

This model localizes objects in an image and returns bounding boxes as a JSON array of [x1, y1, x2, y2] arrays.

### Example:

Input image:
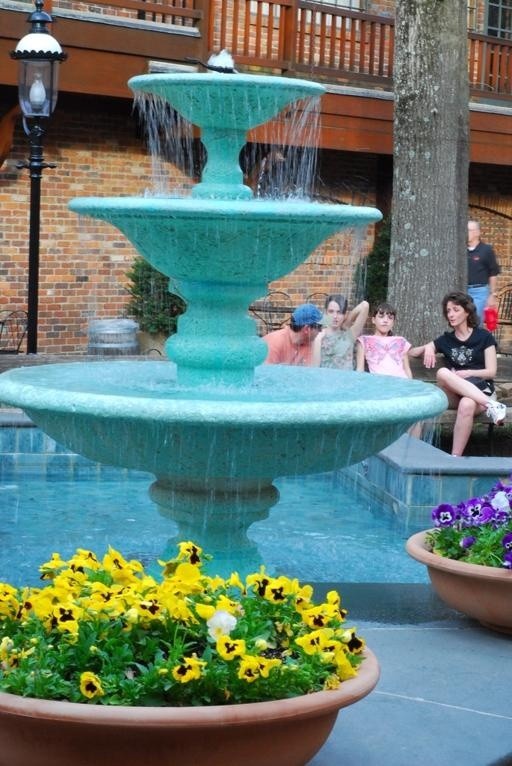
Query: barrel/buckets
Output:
[[87, 314, 139, 356]]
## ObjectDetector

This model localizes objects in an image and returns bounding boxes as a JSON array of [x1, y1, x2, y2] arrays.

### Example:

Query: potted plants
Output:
[[123, 260, 185, 354]]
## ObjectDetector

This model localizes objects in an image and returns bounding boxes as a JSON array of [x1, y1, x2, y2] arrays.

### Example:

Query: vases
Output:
[[0, 647, 381, 766], [406, 527, 512, 638]]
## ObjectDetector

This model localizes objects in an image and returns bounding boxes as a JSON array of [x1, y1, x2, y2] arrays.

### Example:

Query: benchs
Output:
[[352, 358, 512, 457]]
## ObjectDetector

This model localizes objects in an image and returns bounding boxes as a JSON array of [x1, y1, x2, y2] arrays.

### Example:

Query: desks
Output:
[[248, 306, 296, 333]]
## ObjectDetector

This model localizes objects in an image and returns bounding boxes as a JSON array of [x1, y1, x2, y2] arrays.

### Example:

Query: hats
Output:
[[292, 303, 335, 327], [483, 303, 499, 331]]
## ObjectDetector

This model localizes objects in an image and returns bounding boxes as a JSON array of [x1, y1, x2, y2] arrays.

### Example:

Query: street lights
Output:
[[8, 0, 71, 355]]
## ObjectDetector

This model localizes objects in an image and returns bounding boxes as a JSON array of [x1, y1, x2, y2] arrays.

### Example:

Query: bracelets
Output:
[[490, 292, 498, 297]]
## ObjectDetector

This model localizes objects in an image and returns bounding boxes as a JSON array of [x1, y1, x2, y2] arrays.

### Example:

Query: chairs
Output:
[[483, 284, 512, 356], [262, 291, 295, 340], [0, 310, 32, 354], [305, 293, 329, 315], [0, 310, 21, 350]]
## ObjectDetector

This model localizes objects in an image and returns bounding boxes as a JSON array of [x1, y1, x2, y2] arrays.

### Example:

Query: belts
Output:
[[466, 281, 488, 290]]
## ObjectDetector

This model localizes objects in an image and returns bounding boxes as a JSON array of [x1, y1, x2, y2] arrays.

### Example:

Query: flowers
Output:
[[0, 538, 365, 707], [423, 472, 512, 569]]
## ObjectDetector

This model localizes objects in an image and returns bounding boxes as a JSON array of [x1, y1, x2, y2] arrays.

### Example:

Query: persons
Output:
[[467, 220, 500, 328], [260, 304, 334, 366], [356, 303, 422, 440], [311, 294, 369, 370], [411, 292, 507, 456]]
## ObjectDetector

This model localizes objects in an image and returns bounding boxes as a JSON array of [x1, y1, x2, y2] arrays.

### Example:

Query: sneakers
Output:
[[485, 399, 507, 426]]
